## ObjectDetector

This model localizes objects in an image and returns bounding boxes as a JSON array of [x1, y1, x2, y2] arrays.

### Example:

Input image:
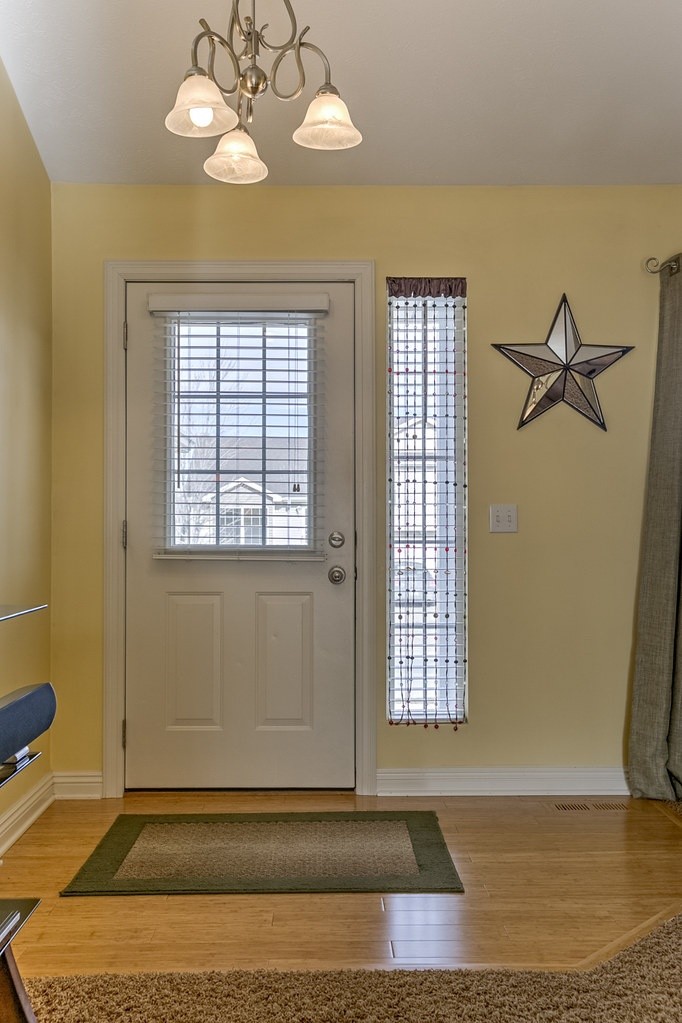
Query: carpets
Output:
[[20, 910, 682, 1023], [58, 809, 465, 895]]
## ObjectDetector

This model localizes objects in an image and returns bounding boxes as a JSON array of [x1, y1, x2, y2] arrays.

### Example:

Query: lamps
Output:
[[164, 0, 361, 183]]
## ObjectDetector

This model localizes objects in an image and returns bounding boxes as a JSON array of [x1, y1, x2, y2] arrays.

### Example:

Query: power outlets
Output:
[[489, 502, 518, 533]]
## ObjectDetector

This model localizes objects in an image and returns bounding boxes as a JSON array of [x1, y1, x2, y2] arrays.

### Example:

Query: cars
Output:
[[394, 559, 440, 605]]
